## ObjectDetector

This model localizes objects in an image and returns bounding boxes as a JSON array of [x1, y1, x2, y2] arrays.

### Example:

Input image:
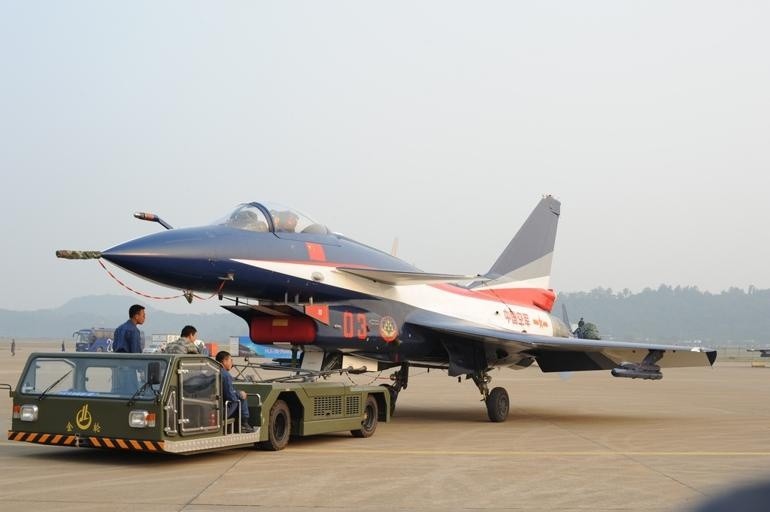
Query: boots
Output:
[[242, 418, 255, 433]]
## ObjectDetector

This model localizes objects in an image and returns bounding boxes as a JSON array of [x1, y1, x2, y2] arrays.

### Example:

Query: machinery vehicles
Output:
[[0, 354, 391, 456]]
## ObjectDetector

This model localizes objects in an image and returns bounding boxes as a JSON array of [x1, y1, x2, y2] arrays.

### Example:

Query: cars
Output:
[[144, 342, 164, 353]]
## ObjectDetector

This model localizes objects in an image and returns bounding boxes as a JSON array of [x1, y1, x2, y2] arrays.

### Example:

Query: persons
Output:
[[216, 351, 254, 433], [10, 339, 15, 356], [112, 304, 146, 393], [165, 325, 201, 354], [578, 317, 584, 328], [61, 341, 65, 352]]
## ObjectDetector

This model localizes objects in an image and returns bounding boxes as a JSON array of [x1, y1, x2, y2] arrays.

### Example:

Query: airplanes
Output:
[[55, 195, 718, 421]]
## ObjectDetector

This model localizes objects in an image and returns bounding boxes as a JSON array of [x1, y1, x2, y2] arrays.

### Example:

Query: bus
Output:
[[73, 327, 144, 353]]
